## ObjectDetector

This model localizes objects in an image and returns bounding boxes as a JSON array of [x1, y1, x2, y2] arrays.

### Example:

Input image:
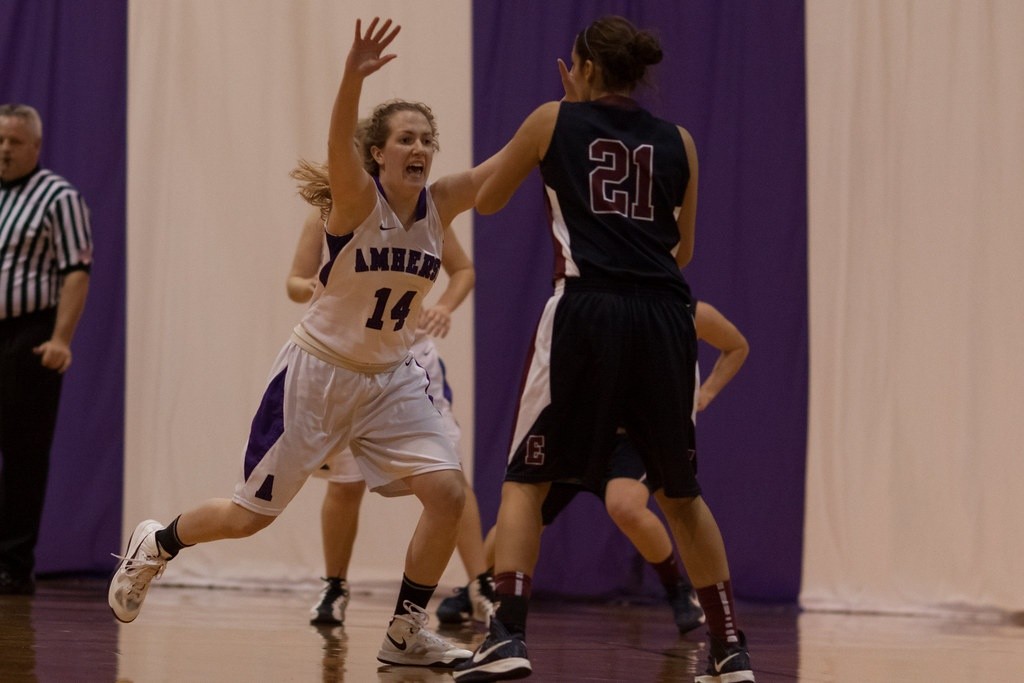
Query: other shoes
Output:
[[0, 571, 36, 595]]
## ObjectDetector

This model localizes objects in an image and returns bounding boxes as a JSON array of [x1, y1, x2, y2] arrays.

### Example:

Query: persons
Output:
[[448, 11, 758, 683], [0, 105, 92, 593], [107, 17, 750, 667]]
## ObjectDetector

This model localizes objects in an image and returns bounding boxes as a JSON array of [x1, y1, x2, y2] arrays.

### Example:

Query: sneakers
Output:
[[310, 577, 348, 626], [376, 599, 474, 667], [467, 575, 498, 624], [437, 586, 474, 628], [107, 520, 179, 623], [451, 619, 531, 683], [666, 583, 707, 634], [693, 630, 756, 683]]
